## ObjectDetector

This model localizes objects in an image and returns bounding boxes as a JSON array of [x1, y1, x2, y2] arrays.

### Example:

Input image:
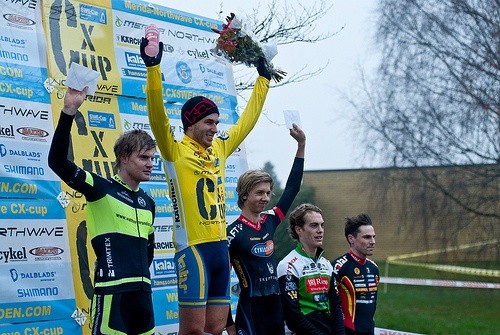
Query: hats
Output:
[[181, 96, 219, 130]]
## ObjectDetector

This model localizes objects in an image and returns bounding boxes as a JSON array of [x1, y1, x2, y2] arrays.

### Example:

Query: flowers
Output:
[[211, 14, 287, 83]]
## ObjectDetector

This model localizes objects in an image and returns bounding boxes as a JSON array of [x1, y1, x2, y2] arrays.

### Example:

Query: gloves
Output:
[[257, 57, 271, 80], [140, 37, 163, 67]]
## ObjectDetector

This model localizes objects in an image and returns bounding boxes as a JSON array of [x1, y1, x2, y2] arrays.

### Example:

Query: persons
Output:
[[275, 203, 342, 335], [48, 86, 157, 335], [329, 213, 382, 335], [139, 36, 271, 335], [225, 122, 305, 335]]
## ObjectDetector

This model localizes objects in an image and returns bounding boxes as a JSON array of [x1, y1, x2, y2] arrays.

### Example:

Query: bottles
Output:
[[145, 24, 160, 57]]
